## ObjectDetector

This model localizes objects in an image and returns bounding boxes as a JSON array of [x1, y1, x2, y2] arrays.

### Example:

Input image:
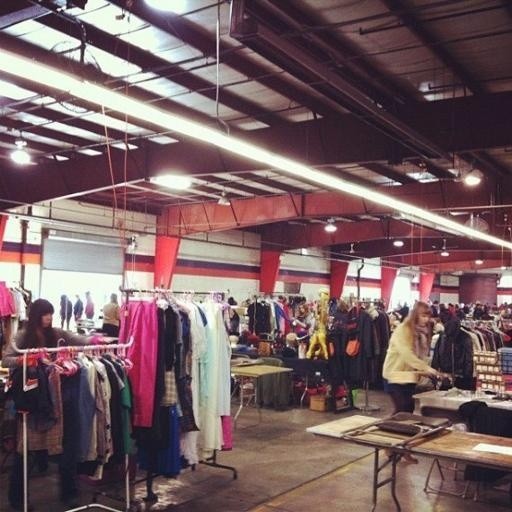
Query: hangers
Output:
[[148, 287, 231, 311], [26, 342, 135, 375]]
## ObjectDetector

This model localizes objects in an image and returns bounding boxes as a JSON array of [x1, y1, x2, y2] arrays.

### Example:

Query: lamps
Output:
[[216, 190, 231, 207]]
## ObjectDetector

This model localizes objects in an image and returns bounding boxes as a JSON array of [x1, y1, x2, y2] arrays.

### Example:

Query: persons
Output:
[[72, 294, 86, 321], [395, 297, 511, 317], [85, 291, 98, 319], [378, 300, 458, 462], [58, 294, 74, 331], [291, 301, 317, 350], [1, 296, 115, 375], [101, 292, 122, 337]]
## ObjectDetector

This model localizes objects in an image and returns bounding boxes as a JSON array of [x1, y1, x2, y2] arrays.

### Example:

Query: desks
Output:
[[230, 365, 294, 431], [306, 391, 512, 511]]
